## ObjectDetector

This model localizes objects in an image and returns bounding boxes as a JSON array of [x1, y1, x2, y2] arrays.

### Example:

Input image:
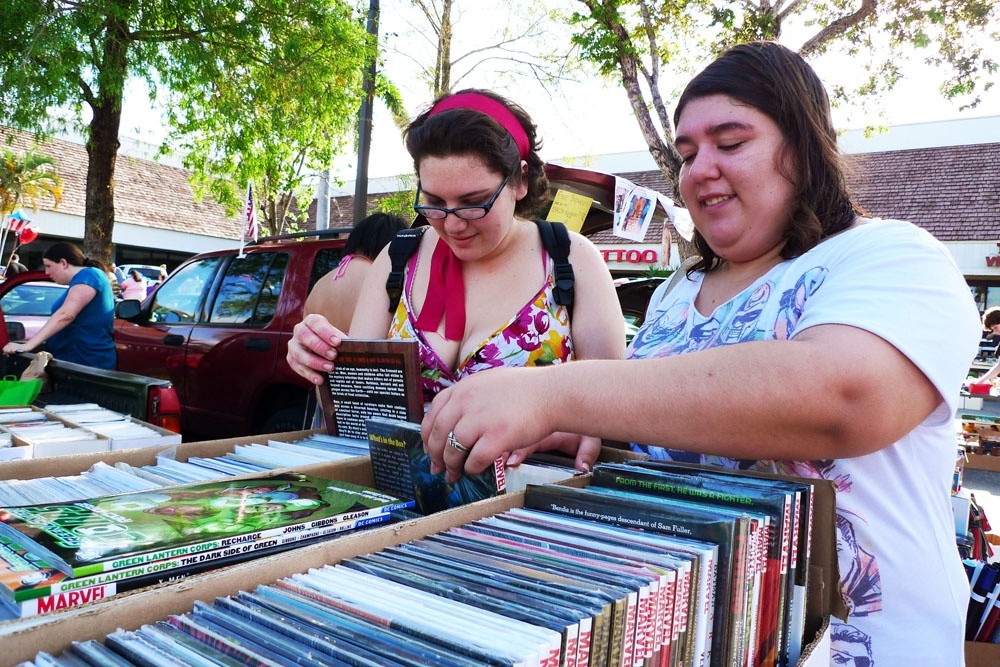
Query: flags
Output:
[[247, 185, 258, 243]]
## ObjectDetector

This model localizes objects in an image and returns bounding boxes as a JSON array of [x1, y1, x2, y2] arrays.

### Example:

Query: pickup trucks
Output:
[[0, 322, 183, 444]]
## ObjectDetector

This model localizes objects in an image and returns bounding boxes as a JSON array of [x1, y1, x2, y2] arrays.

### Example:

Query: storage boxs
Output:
[[1, 425, 33, 461], [963, 452, 1000, 472], [626, 456, 853, 667], [1, 423, 651, 666], [1, 404, 112, 458], [964, 640, 1000, 667], [111, 402, 182, 452]]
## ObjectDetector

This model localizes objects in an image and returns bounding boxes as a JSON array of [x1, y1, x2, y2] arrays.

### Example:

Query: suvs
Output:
[[111, 166, 684, 444]]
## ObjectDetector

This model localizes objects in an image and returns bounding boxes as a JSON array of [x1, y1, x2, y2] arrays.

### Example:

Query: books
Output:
[[0, 402, 815, 667], [317, 339, 424, 441]]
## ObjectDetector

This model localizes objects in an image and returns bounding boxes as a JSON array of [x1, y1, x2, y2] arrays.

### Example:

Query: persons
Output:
[[2, 242, 117, 371], [110, 261, 166, 305], [2, 254, 28, 279], [970, 306, 1000, 385], [419, 42, 984, 667], [285, 95, 627, 414], [303, 212, 409, 334]]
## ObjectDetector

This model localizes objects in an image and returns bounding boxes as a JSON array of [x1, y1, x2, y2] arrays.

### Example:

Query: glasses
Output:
[[414, 176, 509, 220]]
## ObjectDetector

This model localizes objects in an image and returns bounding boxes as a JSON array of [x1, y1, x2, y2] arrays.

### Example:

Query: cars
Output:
[[118, 265, 168, 288], [0, 280, 70, 345]]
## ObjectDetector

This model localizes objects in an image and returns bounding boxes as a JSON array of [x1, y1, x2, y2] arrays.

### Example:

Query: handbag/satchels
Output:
[[0, 375, 44, 406]]
